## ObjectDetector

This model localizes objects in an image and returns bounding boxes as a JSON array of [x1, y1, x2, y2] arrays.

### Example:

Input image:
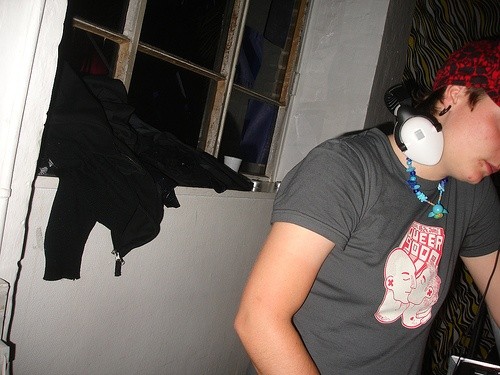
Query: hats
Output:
[[433, 38, 499, 106]]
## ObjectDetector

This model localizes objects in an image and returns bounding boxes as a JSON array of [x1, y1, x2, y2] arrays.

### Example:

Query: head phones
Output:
[[384, 81, 444, 166]]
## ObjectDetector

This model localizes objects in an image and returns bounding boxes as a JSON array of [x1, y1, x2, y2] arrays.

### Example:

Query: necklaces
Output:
[[405, 155, 449, 219]]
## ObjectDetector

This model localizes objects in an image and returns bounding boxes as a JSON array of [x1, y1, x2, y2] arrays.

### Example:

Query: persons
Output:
[[233, 31, 500, 375]]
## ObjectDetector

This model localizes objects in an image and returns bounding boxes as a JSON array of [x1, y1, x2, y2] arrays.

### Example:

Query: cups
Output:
[[224, 155, 242, 173]]
